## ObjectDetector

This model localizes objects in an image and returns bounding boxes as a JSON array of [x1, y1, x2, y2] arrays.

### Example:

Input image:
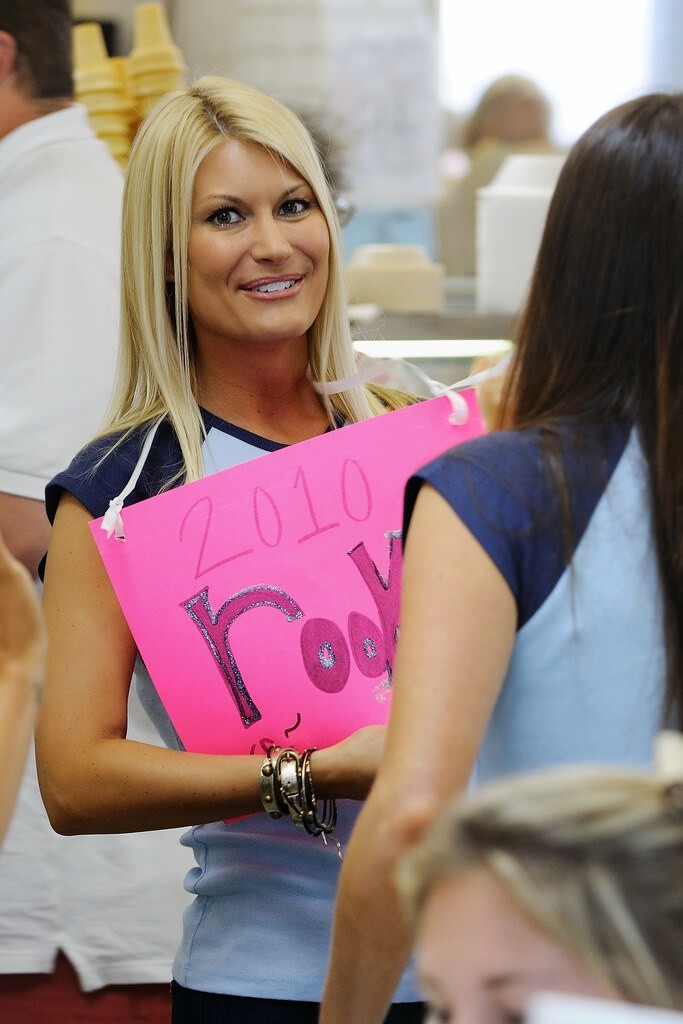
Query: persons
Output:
[[0, 540, 48, 847], [0, 0, 158, 583], [320, 93, 683, 1024], [434, 74, 550, 280], [393, 769, 683, 1024], [37, 74, 473, 1024]]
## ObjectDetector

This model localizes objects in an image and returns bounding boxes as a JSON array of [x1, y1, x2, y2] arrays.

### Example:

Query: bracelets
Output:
[[260, 745, 343, 861]]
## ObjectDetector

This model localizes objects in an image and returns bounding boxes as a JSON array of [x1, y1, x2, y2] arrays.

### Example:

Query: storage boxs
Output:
[[474, 152, 569, 315]]
